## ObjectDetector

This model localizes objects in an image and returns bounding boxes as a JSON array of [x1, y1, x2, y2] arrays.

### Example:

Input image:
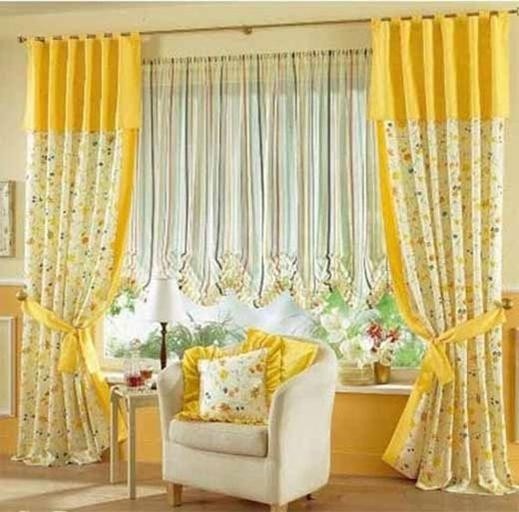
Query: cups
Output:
[[140, 359, 154, 394]]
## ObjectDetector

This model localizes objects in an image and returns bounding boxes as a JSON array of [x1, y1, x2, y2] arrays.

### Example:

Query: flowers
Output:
[[339, 317, 405, 367]]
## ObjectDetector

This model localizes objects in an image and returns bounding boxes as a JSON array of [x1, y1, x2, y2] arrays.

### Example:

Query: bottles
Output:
[[124, 337, 145, 394]]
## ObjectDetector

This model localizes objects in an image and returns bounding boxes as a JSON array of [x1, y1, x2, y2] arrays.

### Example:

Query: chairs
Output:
[[155, 334, 338, 512]]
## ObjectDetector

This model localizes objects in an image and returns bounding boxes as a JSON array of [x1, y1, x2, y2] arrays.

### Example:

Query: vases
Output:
[[341, 357, 391, 386]]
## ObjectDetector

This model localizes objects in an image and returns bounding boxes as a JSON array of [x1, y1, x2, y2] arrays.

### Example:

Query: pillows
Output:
[[175, 324, 323, 426]]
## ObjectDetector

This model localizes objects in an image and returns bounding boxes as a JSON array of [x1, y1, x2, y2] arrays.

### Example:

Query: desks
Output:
[[109, 383, 160, 500]]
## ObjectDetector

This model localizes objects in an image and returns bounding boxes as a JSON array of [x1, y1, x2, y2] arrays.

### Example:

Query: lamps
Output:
[[143, 278, 187, 390]]
